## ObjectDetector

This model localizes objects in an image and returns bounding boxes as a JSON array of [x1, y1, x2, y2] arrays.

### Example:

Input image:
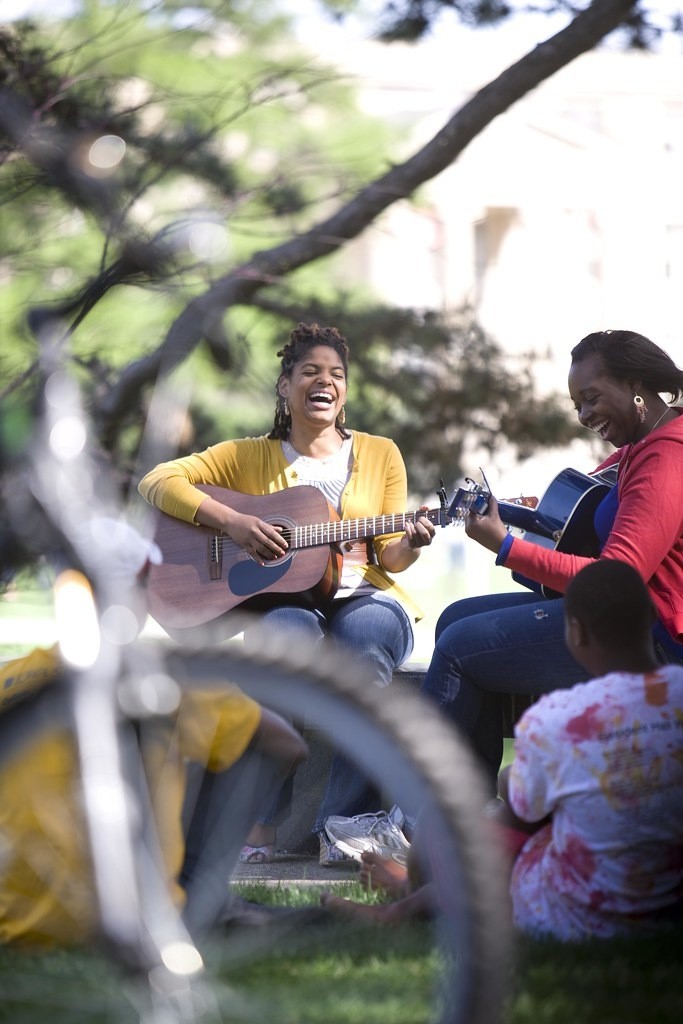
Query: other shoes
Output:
[[238, 842, 275, 864], [317, 829, 360, 866]]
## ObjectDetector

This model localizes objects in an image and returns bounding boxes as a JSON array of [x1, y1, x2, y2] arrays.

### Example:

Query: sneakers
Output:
[[324, 811, 410, 877]]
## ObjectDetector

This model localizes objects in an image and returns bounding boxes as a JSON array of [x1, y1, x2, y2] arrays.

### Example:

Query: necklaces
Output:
[[650, 407, 670, 434]]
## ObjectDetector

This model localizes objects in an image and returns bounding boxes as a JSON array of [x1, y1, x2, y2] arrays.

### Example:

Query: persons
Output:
[[137, 323, 437, 867], [321, 329, 683, 900], [312, 558, 683, 964]]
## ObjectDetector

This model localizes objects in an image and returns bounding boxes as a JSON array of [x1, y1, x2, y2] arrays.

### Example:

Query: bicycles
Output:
[[0, 214, 523, 1023]]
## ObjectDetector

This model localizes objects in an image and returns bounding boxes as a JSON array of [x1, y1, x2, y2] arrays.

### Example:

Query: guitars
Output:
[[143, 482, 539, 650], [446, 465, 621, 602]]
